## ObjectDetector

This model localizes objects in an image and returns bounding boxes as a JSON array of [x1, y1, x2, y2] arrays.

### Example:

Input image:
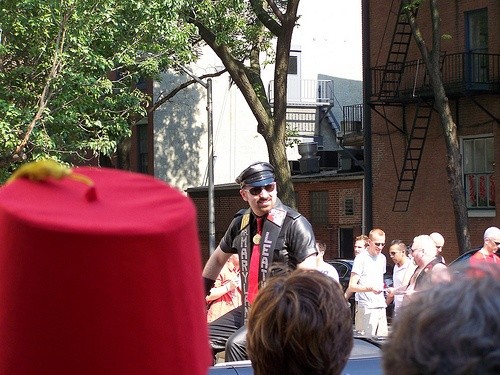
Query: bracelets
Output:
[[225, 284, 229, 292]]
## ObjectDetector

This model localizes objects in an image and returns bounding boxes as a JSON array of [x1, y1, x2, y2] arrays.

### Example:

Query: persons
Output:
[[315, 240, 339, 283], [344, 228, 388, 337], [382, 263, 500, 375], [386, 240, 419, 312], [430, 233, 446, 264], [202, 162, 317, 362], [469, 227, 500, 277], [246, 268, 353, 375], [206, 254, 243, 323], [387, 235, 449, 306]]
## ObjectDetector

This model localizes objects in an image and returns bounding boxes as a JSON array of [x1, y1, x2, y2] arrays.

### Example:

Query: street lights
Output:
[[133, 50, 216, 258]]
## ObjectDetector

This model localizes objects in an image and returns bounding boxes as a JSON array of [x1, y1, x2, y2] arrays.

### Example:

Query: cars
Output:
[[325, 258, 395, 315]]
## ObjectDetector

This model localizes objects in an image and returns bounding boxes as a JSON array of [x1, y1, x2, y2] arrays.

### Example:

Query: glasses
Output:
[[244, 184, 274, 195], [488, 240, 500, 245], [374, 243, 385, 246], [388, 252, 395, 256], [411, 249, 417, 252]]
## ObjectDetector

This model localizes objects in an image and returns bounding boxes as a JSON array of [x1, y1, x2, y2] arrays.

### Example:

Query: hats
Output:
[[235, 162, 275, 186]]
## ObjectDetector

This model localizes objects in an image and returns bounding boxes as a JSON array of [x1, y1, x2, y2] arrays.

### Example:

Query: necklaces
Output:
[[481, 248, 496, 263], [253, 234, 261, 244]]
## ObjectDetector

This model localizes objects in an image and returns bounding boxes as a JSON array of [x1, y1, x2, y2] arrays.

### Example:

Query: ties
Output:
[[247, 218, 262, 305]]
